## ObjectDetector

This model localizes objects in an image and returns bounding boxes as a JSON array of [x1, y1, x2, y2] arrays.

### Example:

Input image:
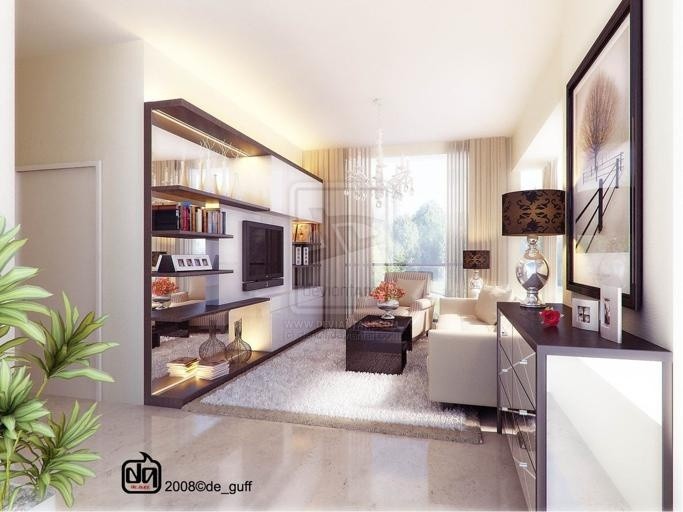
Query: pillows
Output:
[[395, 277, 427, 308], [473, 283, 511, 327]]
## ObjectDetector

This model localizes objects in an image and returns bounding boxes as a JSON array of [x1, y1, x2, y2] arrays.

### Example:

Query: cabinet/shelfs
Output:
[[495, 302, 674, 512], [143, 96, 270, 409]]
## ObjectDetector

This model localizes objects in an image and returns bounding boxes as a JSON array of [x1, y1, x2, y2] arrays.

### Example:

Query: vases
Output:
[[376, 296, 399, 319]]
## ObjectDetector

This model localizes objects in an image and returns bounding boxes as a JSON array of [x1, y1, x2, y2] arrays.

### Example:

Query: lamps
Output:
[[462, 250, 490, 298], [499, 189, 568, 309], [343, 94, 412, 207]]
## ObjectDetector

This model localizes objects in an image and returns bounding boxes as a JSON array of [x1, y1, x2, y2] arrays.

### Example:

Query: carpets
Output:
[[184, 326, 484, 446]]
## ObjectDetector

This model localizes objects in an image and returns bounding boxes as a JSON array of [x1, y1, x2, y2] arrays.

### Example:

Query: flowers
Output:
[[537, 305, 564, 327], [371, 279, 406, 303]]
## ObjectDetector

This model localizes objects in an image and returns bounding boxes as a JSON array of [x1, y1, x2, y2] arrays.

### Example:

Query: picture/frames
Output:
[[562, 0, 643, 312], [571, 297, 598, 330], [599, 284, 622, 345]]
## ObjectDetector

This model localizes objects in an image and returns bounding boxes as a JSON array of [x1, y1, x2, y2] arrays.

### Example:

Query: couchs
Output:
[[425, 296, 496, 412]]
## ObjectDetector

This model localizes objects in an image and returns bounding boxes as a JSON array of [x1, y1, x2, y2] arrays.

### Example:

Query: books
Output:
[[155, 254, 174, 273], [152, 202, 228, 236], [359, 319, 396, 328], [196, 356, 230, 382], [165, 357, 198, 378], [295, 223, 319, 244]]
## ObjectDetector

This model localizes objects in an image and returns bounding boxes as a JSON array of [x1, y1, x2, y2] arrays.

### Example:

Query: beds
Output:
[[344, 314, 412, 374]]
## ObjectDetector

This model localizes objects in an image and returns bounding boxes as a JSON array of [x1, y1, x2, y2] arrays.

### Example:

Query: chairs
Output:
[[353, 270, 434, 343]]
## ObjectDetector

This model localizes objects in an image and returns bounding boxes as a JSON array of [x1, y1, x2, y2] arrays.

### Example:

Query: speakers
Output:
[[243, 279, 283, 291]]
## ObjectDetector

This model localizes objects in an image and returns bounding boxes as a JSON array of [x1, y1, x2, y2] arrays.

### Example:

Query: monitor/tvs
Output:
[[243, 221, 283, 281]]
